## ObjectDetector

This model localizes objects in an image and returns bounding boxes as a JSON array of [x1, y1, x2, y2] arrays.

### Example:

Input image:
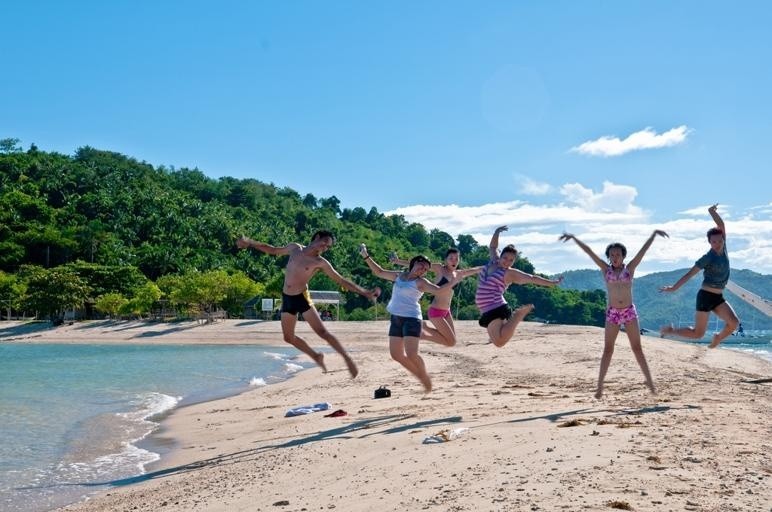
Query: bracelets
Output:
[[364, 255, 370, 260]]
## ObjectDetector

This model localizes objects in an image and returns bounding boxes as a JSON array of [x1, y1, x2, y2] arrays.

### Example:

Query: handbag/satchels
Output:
[[374, 384, 392, 399]]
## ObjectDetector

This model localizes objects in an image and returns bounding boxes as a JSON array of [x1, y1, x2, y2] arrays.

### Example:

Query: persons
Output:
[[560, 230, 669, 399], [641, 327, 645, 334], [272, 309, 282, 321], [659, 203, 739, 348], [390, 249, 485, 346], [475, 226, 562, 347], [318, 307, 333, 321], [361, 243, 463, 399], [237, 230, 380, 378], [735, 323, 744, 337]]
